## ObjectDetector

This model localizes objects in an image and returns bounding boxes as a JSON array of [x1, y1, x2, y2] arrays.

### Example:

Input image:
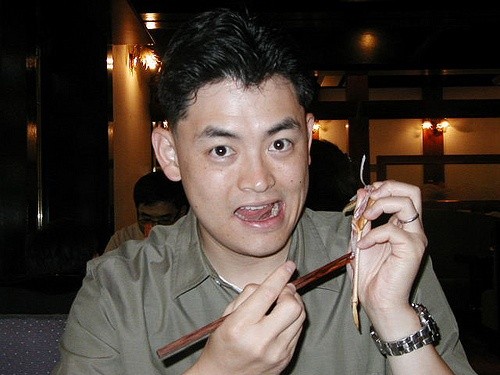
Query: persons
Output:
[[55, 9, 477, 375], [103, 171, 188, 254]]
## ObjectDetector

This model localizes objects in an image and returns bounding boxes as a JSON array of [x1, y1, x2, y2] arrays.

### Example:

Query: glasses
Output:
[[137, 209, 181, 225]]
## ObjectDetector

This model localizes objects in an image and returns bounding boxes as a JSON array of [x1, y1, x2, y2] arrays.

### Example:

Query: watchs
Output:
[[369, 301, 439, 356]]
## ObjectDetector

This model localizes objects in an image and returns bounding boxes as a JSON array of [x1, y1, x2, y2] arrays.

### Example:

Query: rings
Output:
[[398, 213, 419, 224]]
